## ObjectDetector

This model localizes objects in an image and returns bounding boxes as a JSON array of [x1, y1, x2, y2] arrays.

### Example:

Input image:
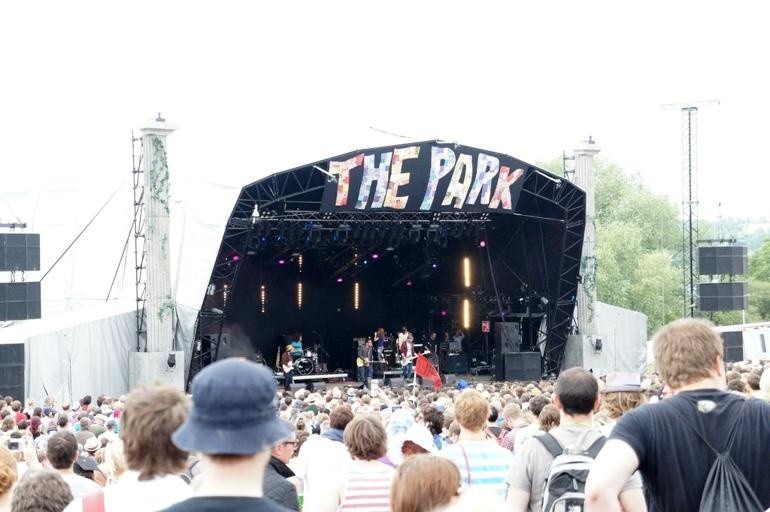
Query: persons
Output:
[[356, 323, 465, 383], [0, 387, 193, 511], [280, 345, 295, 392], [290, 333, 310, 373], [582, 314, 767, 511], [162, 357, 291, 512], [263, 365, 670, 511], [724, 357, 767, 396]]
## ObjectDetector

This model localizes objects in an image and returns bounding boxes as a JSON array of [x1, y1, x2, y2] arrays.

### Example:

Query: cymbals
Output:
[[309, 345, 322, 349]]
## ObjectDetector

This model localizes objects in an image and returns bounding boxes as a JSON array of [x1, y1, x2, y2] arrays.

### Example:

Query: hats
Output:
[[285, 344, 293, 352], [600, 370, 646, 393], [347, 388, 356, 396], [84, 437, 102, 451], [170, 356, 295, 455]]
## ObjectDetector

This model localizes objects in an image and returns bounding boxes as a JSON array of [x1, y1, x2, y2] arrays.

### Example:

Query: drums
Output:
[[295, 358, 313, 375], [304, 350, 312, 358]]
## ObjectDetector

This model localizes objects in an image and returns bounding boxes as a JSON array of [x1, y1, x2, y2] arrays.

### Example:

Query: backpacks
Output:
[[661, 396, 764, 511], [532, 429, 608, 511]]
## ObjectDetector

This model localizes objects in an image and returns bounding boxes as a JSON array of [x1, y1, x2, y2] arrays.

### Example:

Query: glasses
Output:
[[284, 438, 299, 446]]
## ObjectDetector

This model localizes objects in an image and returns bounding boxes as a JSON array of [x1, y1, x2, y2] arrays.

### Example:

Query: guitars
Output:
[[356, 356, 388, 368], [282, 361, 297, 373], [401, 350, 431, 366]]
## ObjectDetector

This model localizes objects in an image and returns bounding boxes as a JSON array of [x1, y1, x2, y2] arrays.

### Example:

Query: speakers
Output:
[[442, 374, 456, 385], [0, 282, 41, 320], [366, 379, 384, 390], [344, 381, 364, 389], [495, 322, 519, 381], [699, 247, 746, 274], [306, 381, 327, 396], [419, 378, 433, 386], [721, 332, 743, 362], [390, 378, 403, 388], [0, 234, 40, 271], [0, 344, 24, 410], [289, 383, 306, 394], [200, 333, 231, 369], [504, 352, 541, 380], [700, 283, 749, 311]]
